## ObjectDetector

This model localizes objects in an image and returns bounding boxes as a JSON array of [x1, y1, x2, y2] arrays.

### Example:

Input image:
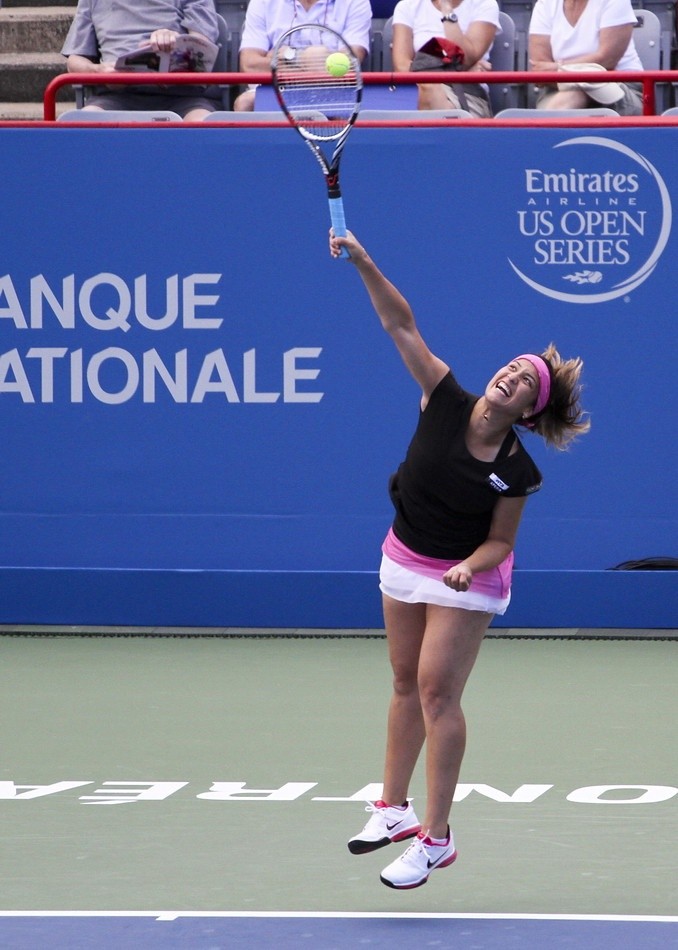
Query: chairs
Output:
[[55, 0, 678, 120]]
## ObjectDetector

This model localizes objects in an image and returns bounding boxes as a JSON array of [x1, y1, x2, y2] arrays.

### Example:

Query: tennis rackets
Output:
[[269, 22, 363, 261]]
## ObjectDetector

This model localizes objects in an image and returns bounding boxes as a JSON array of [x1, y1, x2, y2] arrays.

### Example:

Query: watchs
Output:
[[284, 45, 297, 65], [441, 13, 459, 23]]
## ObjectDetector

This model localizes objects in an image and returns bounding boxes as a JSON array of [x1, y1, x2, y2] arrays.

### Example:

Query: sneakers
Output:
[[379, 820, 456, 890], [347, 795, 422, 855]]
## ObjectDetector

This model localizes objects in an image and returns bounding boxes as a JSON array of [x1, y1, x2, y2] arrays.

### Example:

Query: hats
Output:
[[556, 62, 625, 104], [408, 35, 464, 71]]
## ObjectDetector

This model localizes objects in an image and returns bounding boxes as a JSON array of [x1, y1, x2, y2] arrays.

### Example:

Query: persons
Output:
[[392, 0, 503, 118], [528, 0, 645, 116], [234, 0, 373, 112], [329, 227, 593, 891], [59, 0, 220, 122]]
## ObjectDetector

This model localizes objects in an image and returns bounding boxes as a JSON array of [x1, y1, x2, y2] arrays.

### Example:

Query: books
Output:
[[114, 33, 220, 72]]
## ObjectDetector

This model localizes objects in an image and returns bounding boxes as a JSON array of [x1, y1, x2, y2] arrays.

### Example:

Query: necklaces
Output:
[[477, 404, 503, 431]]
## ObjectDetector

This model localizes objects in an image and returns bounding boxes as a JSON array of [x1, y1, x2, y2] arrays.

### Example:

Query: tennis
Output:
[[325, 52, 351, 76]]
